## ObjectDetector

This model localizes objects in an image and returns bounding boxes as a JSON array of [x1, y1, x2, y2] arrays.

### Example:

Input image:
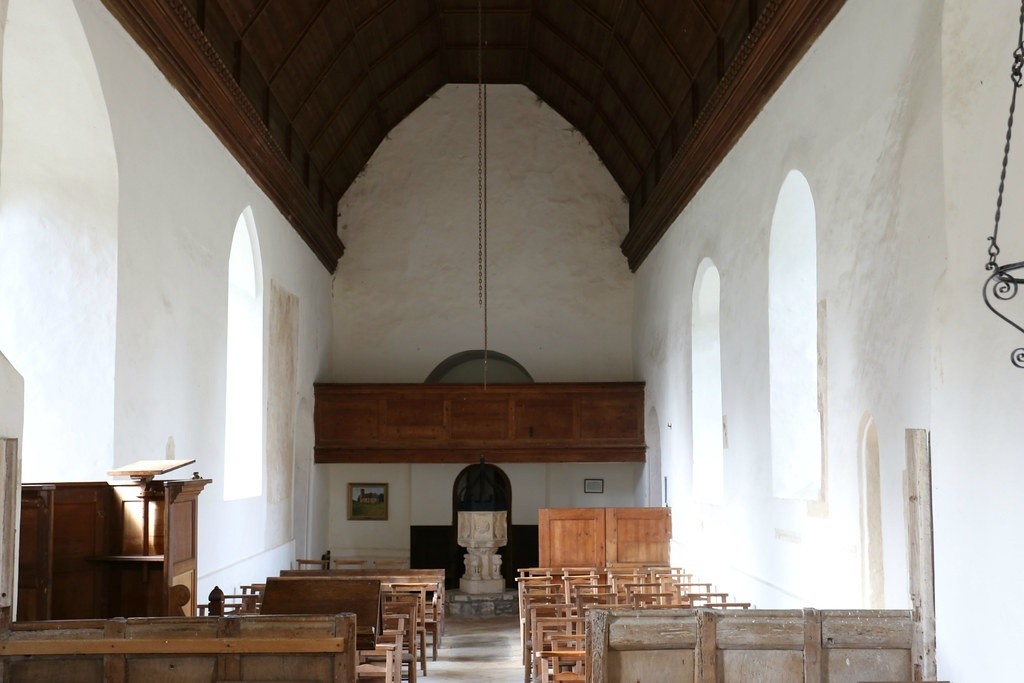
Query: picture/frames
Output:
[[584, 479, 604, 493], [347, 482, 388, 521]]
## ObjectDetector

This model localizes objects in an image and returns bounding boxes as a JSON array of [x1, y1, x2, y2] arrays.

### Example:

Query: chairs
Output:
[[373, 560, 407, 569], [515, 565, 751, 683], [197, 569, 445, 683], [296, 559, 329, 569], [334, 559, 367, 569]]
